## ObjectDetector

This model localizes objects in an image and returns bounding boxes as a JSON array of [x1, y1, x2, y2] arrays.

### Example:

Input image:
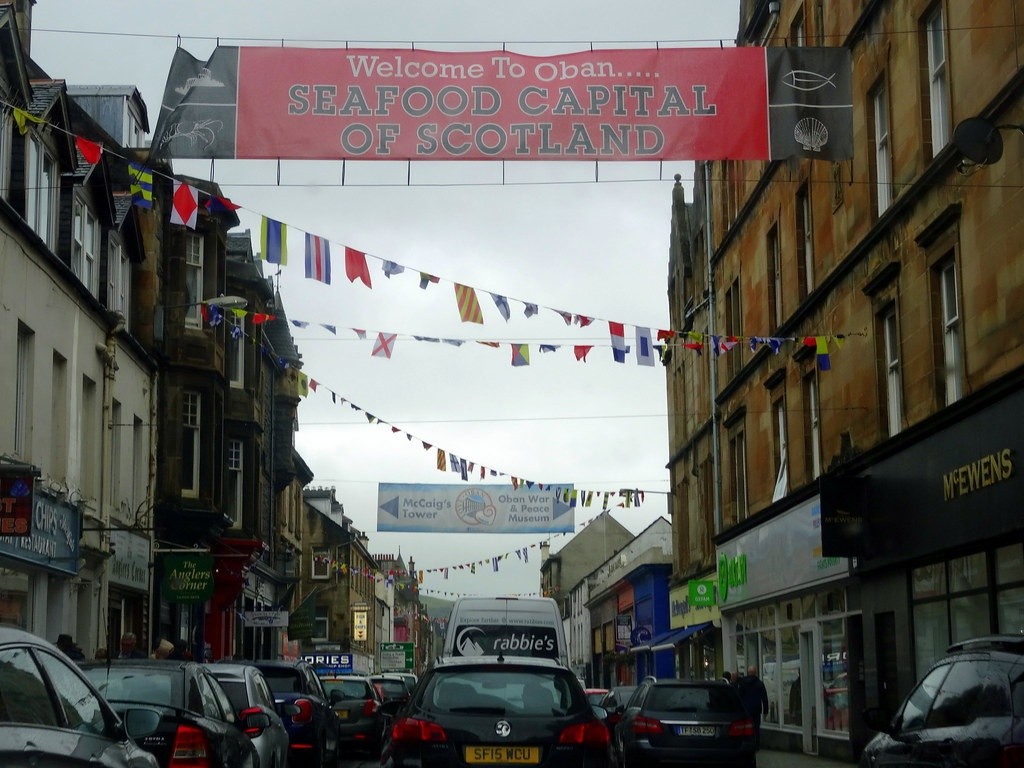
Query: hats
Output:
[[53, 634, 78, 646]]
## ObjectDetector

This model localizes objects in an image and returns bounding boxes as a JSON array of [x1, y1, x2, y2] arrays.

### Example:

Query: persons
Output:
[[789, 669, 803, 726], [721, 665, 770, 752], [148, 637, 193, 662], [55, 634, 85, 661], [112, 630, 149, 659]]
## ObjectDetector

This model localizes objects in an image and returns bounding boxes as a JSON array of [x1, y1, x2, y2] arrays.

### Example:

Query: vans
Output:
[[433, 594, 567, 708]]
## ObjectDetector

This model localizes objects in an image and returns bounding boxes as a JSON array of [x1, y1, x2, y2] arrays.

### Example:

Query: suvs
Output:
[[613, 677, 756, 768], [379, 655, 621, 768], [857, 633, 1024, 768]]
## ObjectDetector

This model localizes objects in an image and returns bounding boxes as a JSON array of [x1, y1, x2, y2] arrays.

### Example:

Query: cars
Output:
[[320, 675, 390, 761], [0, 621, 161, 768], [71, 658, 270, 768], [214, 657, 345, 768], [584, 688, 610, 707], [201, 662, 302, 768], [381, 672, 420, 693], [598, 685, 640, 735], [368, 674, 411, 716]]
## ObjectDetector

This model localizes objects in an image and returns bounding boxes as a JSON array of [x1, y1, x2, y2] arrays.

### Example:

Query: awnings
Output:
[[629, 627, 683, 652], [651, 621, 713, 651]]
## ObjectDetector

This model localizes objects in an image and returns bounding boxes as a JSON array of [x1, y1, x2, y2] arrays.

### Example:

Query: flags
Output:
[[383, 258, 440, 290], [260, 215, 289, 264], [395, 582, 536, 597], [344, 246, 372, 287], [304, 232, 331, 285], [492, 293, 511, 321], [170, 179, 242, 231], [315, 503, 626, 588], [412, 612, 449, 624], [455, 283, 484, 323], [195, 301, 847, 507], [12, 107, 154, 211]]
